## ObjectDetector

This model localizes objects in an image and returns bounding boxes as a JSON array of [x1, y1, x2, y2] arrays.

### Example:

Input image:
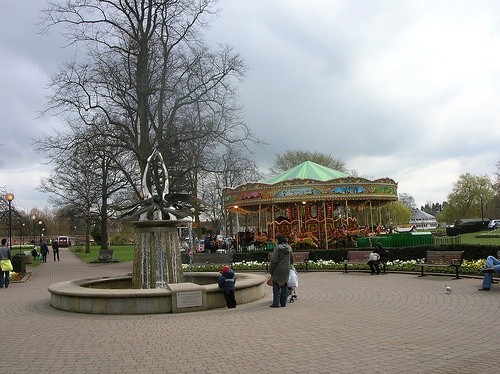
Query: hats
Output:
[[223, 265, 230, 272]]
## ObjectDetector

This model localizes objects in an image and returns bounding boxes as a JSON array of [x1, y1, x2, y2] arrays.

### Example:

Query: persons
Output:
[[218, 266, 236, 308], [52, 241, 60, 262], [31, 249, 38, 260], [478, 256, 500, 291], [367, 242, 386, 275], [0, 239, 11, 288], [41, 242, 49, 263], [268, 236, 294, 307], [204, 233, 213, 254]]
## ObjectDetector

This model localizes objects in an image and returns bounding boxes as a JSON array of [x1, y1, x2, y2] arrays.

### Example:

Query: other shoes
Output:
[[0, 284, 3, 288], [281, 303, 286, 307], [371, 270, 375, 274], [269, 305, 279, 307], [376, 271, 381, 275], [478, 286, 490, 291], [482, 268, 493, 273], [5, 284, 8, 288]]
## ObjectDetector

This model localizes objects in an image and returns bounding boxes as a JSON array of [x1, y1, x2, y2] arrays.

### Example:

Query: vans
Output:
[[57, 236, 69, 247]]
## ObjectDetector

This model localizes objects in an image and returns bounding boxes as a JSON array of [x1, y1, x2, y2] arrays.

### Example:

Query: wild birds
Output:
[[444, 284, 452, 293]]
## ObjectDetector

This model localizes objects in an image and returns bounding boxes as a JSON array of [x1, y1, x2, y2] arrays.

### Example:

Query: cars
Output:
[[487, 219, 500, 230]]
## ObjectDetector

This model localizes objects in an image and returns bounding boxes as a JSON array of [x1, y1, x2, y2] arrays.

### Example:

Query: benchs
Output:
[[191, 253, 234, 271], [265, 252, 311, 274], [412, 250, 465, 281], [339, 250, 389, 275]]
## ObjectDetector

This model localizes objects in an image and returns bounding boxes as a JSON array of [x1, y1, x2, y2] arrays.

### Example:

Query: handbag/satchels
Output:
[[267, 276, 274, 286], [287, 269, 299, 287], [370, 253, 380, 261], [0, 259, 13, 271]]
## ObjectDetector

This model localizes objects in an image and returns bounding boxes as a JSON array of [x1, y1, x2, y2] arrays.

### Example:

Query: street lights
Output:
[[234, 205, 238, 252], [5, 193, 14, 249], [22, 223, 25, 245], [32, 214, 36, 247], [42, 228, 45, 235], [303, 201, 306, 232], [38, 220, 42, 243]]
